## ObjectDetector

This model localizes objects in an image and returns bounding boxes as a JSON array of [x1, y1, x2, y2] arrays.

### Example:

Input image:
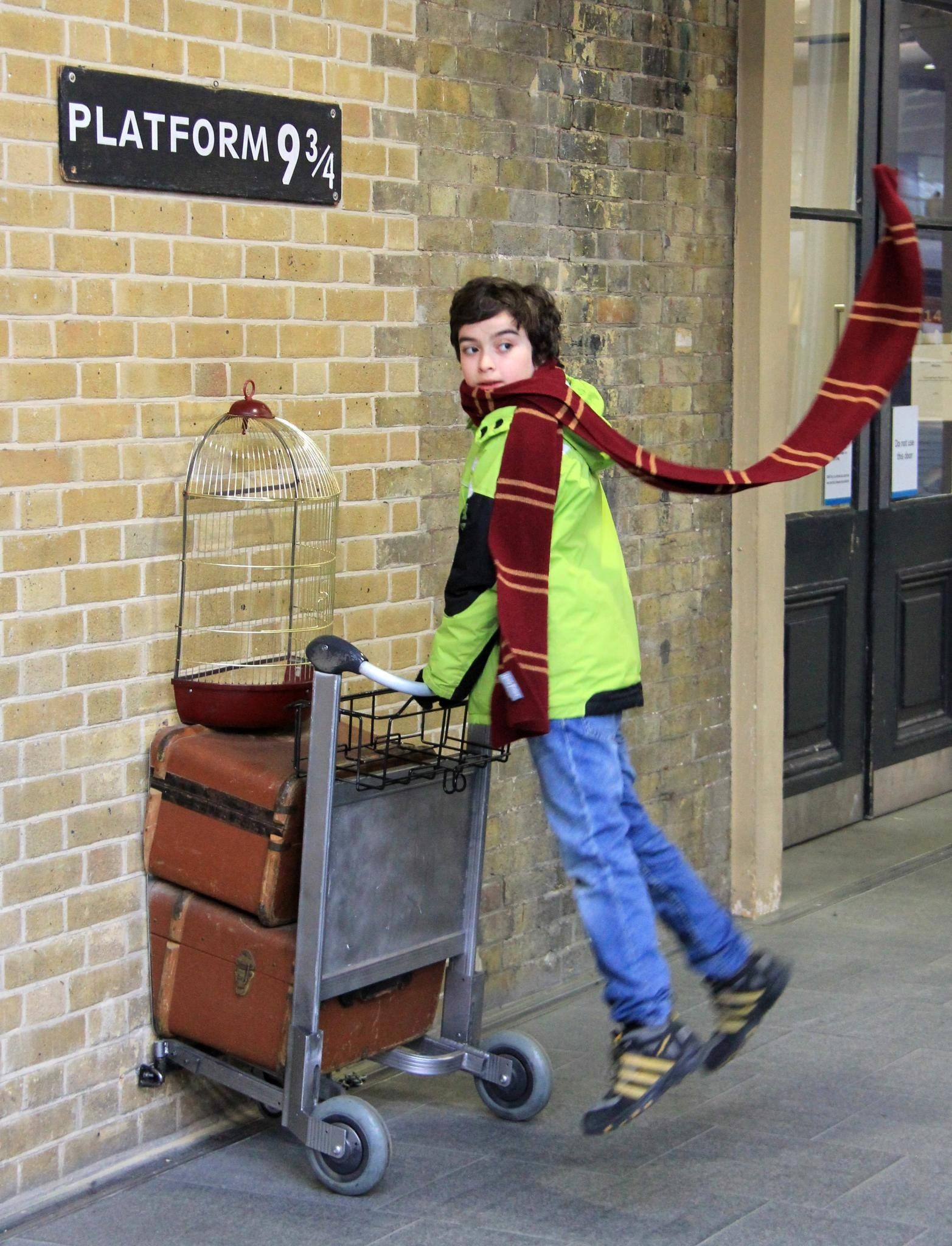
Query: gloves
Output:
[[414, 666, 460, 708]]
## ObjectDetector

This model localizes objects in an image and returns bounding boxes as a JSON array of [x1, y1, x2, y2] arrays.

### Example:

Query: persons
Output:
[[410, 276, 790, 1143]]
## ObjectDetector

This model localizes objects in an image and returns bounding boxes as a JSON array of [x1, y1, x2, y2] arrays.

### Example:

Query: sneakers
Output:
[[701, 948, 793, 1074], [581, 1008, 707, 1136]]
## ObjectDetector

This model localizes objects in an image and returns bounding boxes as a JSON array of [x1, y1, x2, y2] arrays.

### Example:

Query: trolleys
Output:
[[136, 635, 555, 1196]]
